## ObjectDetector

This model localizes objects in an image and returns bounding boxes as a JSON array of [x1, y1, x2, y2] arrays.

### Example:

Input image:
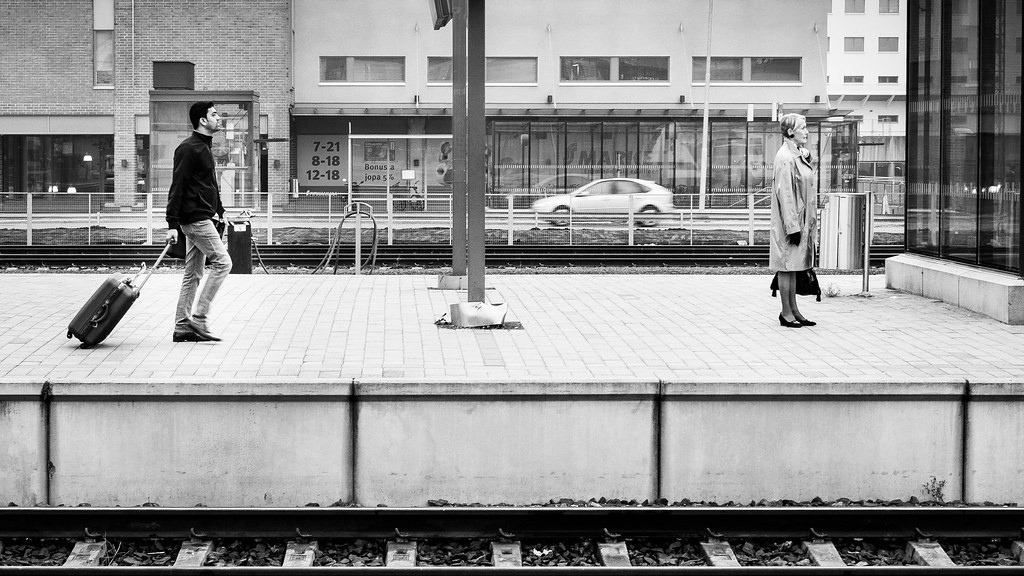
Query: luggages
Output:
[[67, 236, 174, 349]]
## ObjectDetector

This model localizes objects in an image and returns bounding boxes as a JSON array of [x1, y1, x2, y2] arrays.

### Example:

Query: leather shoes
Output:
[[186, 322, 221, 342], [172, 332, 211, 342]]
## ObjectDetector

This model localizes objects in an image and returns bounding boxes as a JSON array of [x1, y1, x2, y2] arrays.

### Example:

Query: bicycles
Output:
[[389, 182, 407, 211], [409, 180, 425, 211]]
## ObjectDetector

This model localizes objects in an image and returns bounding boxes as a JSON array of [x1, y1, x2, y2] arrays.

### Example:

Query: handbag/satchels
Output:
[[770, 266, 822, 302]]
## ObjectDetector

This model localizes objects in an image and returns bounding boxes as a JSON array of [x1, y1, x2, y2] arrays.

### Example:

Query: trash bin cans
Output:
[[818, 193, 866, 270], [228, 220, 253, 274]]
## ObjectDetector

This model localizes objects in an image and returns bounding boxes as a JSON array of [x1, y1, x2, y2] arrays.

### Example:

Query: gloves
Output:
[[789, 232, 801, 246]]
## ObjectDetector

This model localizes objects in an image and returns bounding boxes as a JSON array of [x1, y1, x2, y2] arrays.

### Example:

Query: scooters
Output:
[[341, 178, 364, 211]]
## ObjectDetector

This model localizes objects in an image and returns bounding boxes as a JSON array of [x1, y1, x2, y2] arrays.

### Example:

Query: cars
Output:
[[533, 177, 675, 227], [505, 172, 596, 207]]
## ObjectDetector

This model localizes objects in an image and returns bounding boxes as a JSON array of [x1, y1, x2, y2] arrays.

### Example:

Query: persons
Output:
[[166, 101, 233, 341], [769, 113, 818, 328]]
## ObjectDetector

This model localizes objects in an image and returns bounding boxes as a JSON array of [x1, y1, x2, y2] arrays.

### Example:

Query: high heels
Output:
[[794, 316, 817, 326], [779, 312, 802, 328]]
